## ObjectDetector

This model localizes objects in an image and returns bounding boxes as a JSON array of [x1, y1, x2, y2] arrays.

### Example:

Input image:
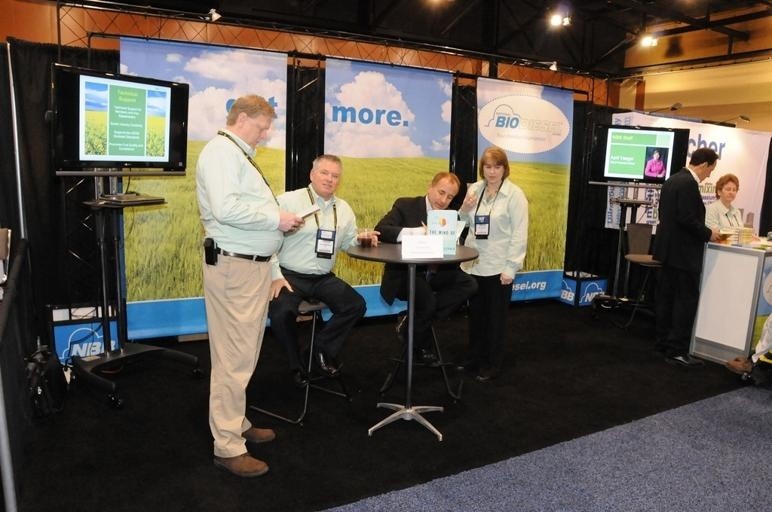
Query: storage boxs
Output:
[[556, 272, 608, 307], [46, 301, 119, 364]]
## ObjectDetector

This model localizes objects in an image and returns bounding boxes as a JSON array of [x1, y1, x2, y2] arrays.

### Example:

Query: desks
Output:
[[347, 244, 479, 441], [689, 237, 772, 380]]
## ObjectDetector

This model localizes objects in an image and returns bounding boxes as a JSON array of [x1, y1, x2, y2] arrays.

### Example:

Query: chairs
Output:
[[622, 223, 655, 300]]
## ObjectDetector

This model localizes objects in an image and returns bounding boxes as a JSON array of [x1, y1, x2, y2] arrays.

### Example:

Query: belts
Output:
[[216, 247, 272, 262]]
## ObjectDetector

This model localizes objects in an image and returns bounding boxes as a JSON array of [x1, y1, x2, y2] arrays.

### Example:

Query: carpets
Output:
[[320, 384, 772, 511]]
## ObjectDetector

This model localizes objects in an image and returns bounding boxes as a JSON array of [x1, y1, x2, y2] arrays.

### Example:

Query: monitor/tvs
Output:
[[588, 123, 690, 205], [50, 62, 189, 205]]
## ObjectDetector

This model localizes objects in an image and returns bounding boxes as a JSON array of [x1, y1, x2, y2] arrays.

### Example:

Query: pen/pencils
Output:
[[421, 220, 426, 227]]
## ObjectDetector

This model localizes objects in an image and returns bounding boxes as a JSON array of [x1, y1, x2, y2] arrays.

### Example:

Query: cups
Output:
[[358, 227, 374, 248]]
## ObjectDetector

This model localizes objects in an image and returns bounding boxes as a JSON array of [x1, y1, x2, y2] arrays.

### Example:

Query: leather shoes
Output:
[[725, 358, 765, 384], [291, 366, 310, 388], [472, 366, 497, 383], [314, 341, 342, 377], [395, 309, 408, 344], [213, 452, 269, 478], [482, 377, 484, 378], [409, 342, 436, 361], [454, 357, 475, 372], [242, 427, 276, 443], [664, 351, 706, 370]]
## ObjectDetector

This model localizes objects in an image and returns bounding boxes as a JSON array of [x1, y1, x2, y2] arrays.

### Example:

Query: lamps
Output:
[[207, 7, 221, 23], [548, 60, 558, 71]]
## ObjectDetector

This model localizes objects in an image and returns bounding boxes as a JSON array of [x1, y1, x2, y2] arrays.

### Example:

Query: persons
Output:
[[270, 154, 381, 389], [645, 150, 666, 178], [458, 147, 529, 380], [194, 95, 305, 478], [652, 147, 723, 368], [725, 313, 772, 374], [375, 171, 479, 365], [705, 174, 744, 230]]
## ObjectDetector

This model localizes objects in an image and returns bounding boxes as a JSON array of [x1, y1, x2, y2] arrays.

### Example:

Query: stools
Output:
[[248, 300, 353, 425]]
